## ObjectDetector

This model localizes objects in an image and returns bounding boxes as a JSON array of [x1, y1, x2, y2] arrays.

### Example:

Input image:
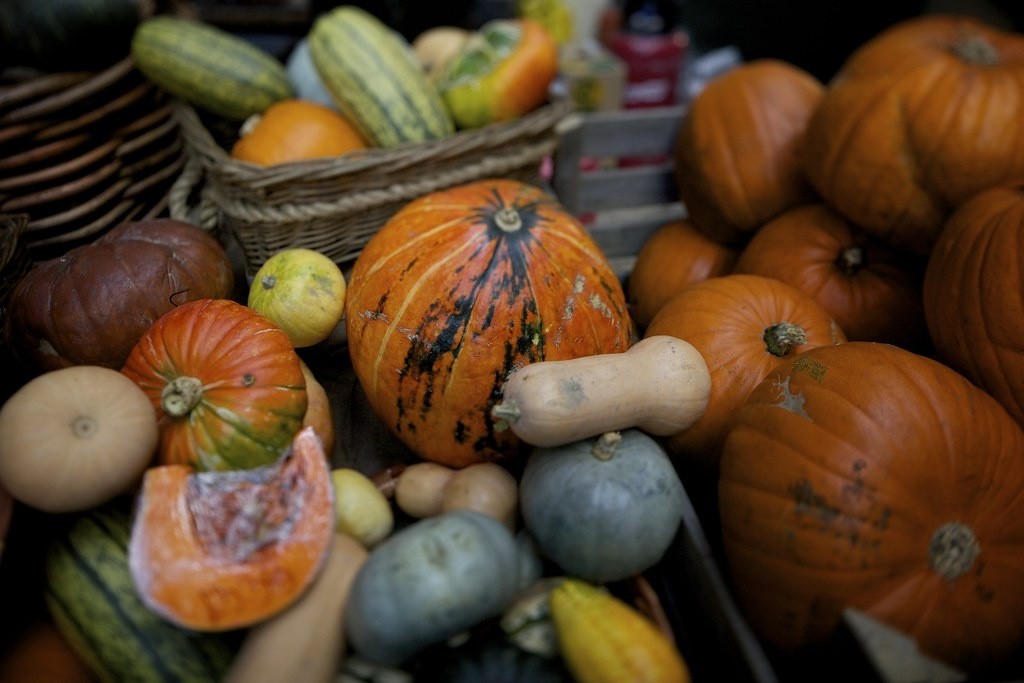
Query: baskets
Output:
[[164, 68, 570, 307]]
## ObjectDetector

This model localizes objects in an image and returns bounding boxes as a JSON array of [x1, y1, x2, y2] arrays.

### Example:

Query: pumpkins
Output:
[[0, 1, 1024, 683]]
